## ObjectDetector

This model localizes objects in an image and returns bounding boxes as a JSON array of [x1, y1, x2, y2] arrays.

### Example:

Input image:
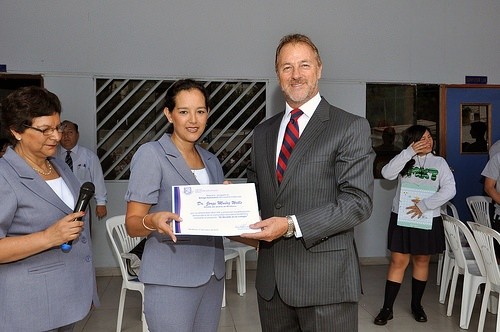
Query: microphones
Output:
[[61, 182, 95, 253]]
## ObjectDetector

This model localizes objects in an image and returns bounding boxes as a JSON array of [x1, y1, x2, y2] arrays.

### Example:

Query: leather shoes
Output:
[[374, 308, 393, 326], [412, 304, 428, 323]]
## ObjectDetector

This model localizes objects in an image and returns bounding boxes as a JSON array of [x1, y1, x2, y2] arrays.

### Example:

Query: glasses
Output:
[[24, 125, 65, 135]]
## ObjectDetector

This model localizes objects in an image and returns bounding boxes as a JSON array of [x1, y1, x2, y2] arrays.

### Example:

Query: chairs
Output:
[[221, 238, 259, 307], [425, 195, 500, 332], [106, 215, 150, 332]]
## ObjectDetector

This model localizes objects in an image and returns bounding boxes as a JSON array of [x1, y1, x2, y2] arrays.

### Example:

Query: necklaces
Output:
[[417, 153, 427, 177], [32, 159, 52, 175]]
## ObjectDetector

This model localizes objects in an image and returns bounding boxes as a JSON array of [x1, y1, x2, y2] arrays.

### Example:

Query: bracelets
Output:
[[142, 214, 157, 231]]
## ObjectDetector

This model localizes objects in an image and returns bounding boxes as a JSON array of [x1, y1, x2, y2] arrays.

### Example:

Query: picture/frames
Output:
[[459, 103, 492, 155]]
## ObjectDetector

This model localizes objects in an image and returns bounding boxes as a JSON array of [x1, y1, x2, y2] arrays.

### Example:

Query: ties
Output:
[[65, 151, 73, 173], [275, 110, 303, 185]]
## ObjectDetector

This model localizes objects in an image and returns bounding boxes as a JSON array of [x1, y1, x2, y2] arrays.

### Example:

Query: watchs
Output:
[[283, 216, 295, 238]]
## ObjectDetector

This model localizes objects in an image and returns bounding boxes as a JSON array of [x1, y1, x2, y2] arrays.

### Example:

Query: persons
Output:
[[463, 121, 488, 151], [0, 86, 101, 332], [374, 125, 457, 325], [481, 151, 500, 266], [0, 138, 11, 158], [125, 78, 226, 332], [48, 120, 108, 239], [374, 127, 404, 179], [223, 34, 376, 332], [96, 132, 117, 181]]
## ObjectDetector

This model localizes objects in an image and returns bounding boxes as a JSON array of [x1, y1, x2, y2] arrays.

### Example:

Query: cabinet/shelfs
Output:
[[0, 73, 44, 159]]
